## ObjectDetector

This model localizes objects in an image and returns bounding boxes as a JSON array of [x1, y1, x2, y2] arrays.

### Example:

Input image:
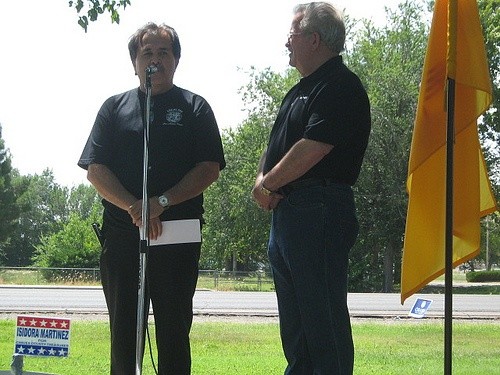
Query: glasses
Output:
[[287, 32, 314, 39]]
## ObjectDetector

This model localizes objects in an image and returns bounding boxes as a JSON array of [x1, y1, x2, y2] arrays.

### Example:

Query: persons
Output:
[[251, 2, 372, 375], [77, 23, 226, 375]]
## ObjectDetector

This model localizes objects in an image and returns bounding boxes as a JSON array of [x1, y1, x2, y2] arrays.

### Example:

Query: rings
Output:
[[140, 217, 142, 222], [259, 203, 262, 208]]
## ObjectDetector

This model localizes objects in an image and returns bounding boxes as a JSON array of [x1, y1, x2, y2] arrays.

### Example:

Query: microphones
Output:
[[144, 64, 158, 73]]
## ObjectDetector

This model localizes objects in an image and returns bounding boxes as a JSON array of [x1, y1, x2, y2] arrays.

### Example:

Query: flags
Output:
[[401, 0, 499, 305]]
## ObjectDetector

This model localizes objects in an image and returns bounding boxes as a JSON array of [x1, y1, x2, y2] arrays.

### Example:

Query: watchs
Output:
[[157, 193, 169, 211], [259, 179, 280, 196]]
